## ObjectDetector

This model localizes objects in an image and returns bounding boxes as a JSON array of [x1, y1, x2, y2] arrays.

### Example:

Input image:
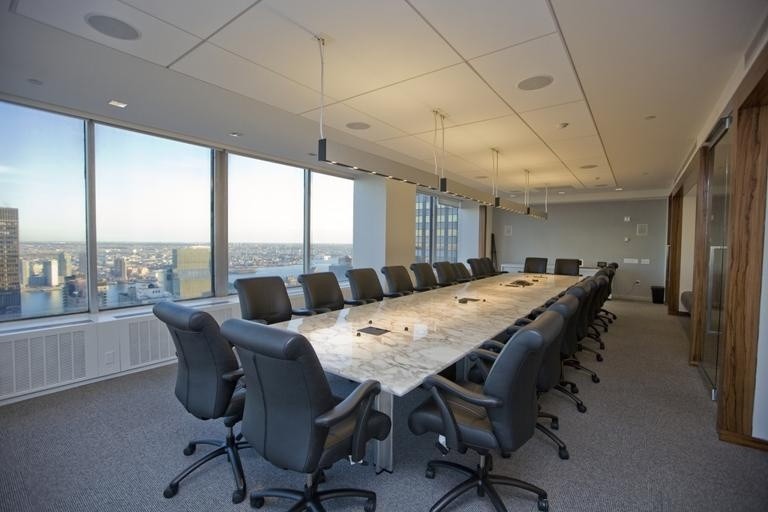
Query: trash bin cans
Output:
[[650, 286, 664, 304]]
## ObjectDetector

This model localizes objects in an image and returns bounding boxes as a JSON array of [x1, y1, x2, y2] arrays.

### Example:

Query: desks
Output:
[[153, 257, 619, 512]]
